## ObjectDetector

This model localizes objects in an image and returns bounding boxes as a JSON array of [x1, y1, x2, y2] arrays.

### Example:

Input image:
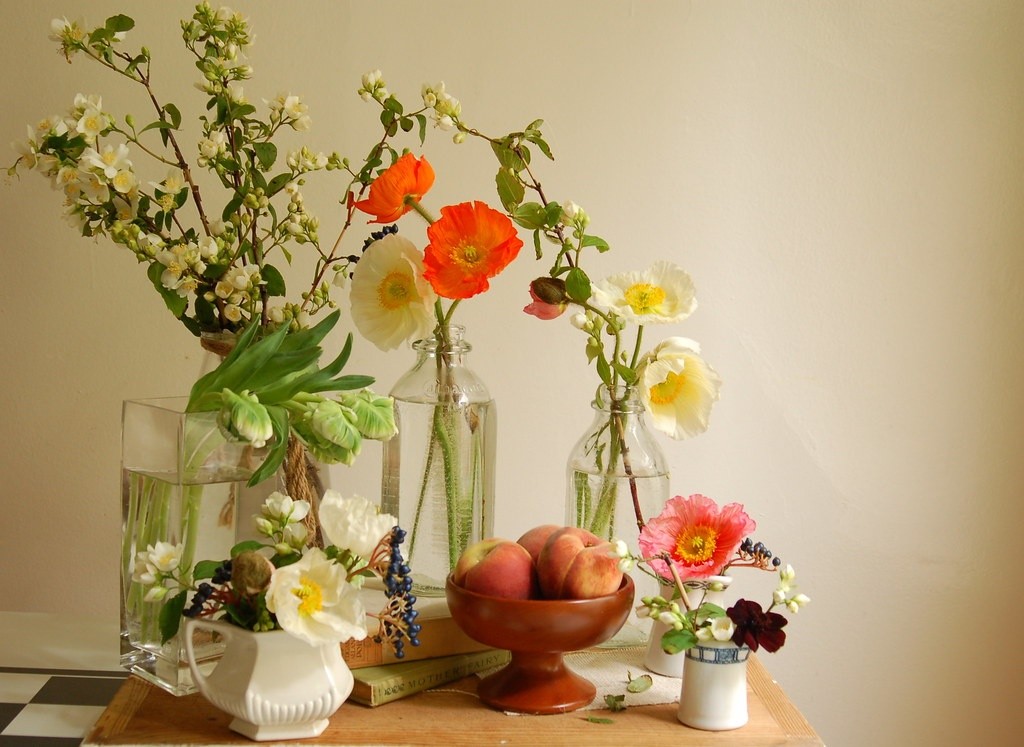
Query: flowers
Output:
[[6, 2, 465, 341], [634, 494, 810, 655], [184, 308, 400, 487], [419, 80, 722, 438], [129, 488, 422, 659], [349, 149, 525, 350]]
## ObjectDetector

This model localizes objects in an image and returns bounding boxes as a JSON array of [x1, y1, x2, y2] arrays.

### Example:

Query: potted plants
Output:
[[119, 394, 329, 695]]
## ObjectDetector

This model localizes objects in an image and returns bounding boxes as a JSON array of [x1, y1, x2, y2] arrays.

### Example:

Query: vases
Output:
[[194, 336, 327, 548], [678, 640, 749, 731], [380, 323, 498, 599], [566, 384, 672, 648], [642, 577, 707, 678], [184, 620, 357, 740]]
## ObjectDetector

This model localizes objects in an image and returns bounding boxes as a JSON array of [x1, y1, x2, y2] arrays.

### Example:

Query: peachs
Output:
[[536, 526, 624, 602], [452, 538, 539, 600], [515, 524, 562, 562]]
